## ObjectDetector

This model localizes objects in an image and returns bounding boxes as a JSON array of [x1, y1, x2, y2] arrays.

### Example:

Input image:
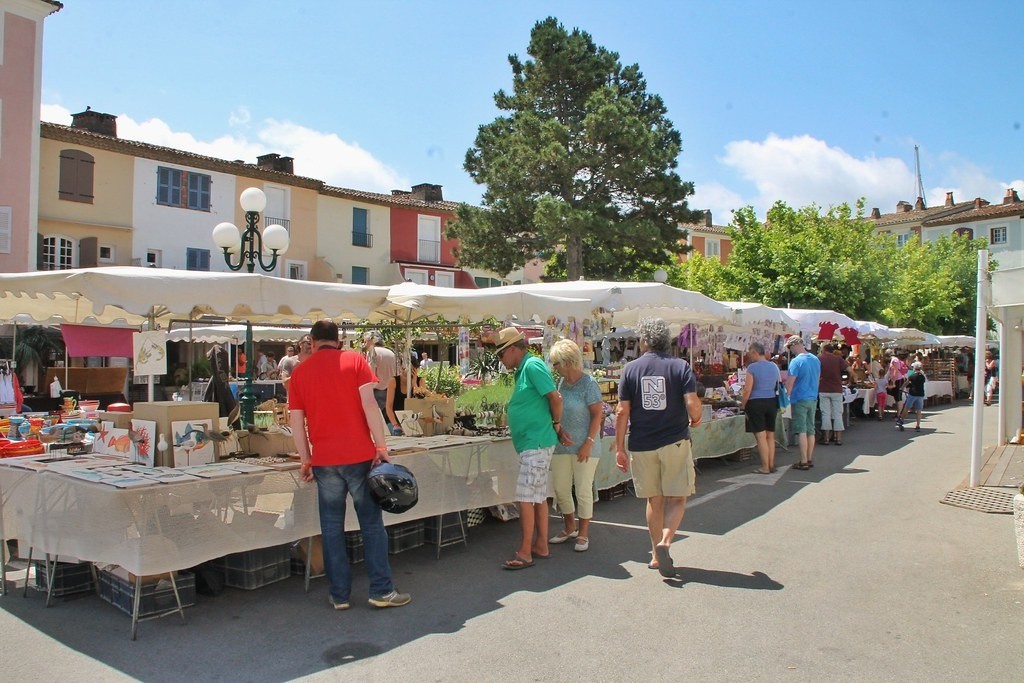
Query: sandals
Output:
[[793, 461, 809, 470], [808, 460, 814, 467]]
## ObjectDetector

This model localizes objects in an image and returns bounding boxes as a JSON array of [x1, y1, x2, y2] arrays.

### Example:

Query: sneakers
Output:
[[368, 590, 412, 607], [328, 592, 350, 609]]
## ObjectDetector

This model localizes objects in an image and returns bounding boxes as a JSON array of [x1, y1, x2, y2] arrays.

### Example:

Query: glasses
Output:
[[787, 344, 796, 349], [300, 340, 311, 345], [553, 361, 560, 369], [498, 344, 513, 357]]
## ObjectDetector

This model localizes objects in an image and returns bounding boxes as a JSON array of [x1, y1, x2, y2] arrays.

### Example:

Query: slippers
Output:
[[751, 467, 770, 474], [655, 544, 676, 577], [531, 549, 552, 559], [500, 553, 535, 569], [648, 560, 659, 569], [770, 466, 777, 473]]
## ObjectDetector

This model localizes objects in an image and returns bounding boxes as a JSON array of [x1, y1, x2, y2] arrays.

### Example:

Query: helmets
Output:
[[367, 463, 418, 513]]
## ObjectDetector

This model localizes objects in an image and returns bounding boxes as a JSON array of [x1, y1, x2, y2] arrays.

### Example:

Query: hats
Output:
[[783, 334, 803, 347], [911, 361, 923, 372], [493, 327, 525, 355]]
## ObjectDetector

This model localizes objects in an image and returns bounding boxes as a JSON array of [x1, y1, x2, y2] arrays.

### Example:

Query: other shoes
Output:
[[816, 392, 994, 448]]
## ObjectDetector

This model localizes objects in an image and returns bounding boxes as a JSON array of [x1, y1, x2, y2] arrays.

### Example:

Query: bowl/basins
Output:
[[7, 400, 105, 440]]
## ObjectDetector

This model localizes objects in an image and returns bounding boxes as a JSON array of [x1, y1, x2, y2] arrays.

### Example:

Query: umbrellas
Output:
[[483, 266, 975, 351], [1, 265, 389, 402], [332, 280, 592, 398]]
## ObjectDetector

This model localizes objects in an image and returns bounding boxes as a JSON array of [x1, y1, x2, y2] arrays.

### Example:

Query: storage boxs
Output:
[[35, 560, 98, 596], [99, 411, 134, 442], [92, 559, 197, 621], [343, 530, 366, 565], [385, 518, 426, 554], [133, 400, 220, 468], [404, 397, 455, 436], [596, 472, 627, 501], [711, 439, 752, 462], [421, 499, 467, 544], [191, 541, 291, 590], [236, 427, 299, 458], [281, 526, 311, 577]]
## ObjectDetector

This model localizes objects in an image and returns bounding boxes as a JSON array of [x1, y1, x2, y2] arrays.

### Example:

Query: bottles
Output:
[[606, 361, 623, 380]]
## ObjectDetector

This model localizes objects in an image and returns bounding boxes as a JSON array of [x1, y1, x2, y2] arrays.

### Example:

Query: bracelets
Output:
[[552, 419, 560, 424], [586, 436, 595, 443], [300, 455, 311, 462], [691, 417, 702, 427], [375, 447, 388, 451]]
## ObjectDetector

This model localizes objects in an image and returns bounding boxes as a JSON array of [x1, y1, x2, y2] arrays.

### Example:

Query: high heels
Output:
[[548, 529, 579, 543], [574, 535, 589, 551]]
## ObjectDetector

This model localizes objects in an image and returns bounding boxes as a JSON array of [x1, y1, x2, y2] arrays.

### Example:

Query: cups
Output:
[[59, 396, 77, 411]]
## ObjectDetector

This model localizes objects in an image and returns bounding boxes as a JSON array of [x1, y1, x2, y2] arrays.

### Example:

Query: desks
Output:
[[0, 378, 953, 642]]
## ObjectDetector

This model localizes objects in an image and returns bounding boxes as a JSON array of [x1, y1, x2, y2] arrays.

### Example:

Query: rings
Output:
[[561, 442, 565, 446]]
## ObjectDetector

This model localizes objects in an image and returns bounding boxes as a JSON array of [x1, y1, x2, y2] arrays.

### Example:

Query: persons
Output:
[[234, 333, 435, 437], [492, 327, 573, 570], [614, 317, 703, 579], [547, 339, 604, 551], [740, 343, 782, 474], [819, 340, 999, 446], [784, 335, 821, 471], [287, 321, 419, 610]]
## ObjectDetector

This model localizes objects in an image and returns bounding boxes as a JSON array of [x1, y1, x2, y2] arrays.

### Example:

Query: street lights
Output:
[[212, 186, 290, 432]]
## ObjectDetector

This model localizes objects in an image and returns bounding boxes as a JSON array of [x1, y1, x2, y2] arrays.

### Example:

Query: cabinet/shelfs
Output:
[[933, 357, 956, 402]]
